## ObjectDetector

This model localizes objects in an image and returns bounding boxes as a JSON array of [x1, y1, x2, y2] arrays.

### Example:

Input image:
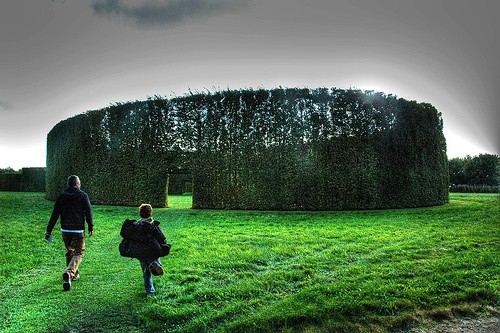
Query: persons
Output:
[[45, 175, 94, 291], [119, 203, 172, 297]]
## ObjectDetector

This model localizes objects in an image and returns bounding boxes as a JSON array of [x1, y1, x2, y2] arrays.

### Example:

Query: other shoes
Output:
[[152, 262, 164, 275], [62, 273, 72, 290], [145, 291, 156, 297], [73, 272, 79, 280]]
